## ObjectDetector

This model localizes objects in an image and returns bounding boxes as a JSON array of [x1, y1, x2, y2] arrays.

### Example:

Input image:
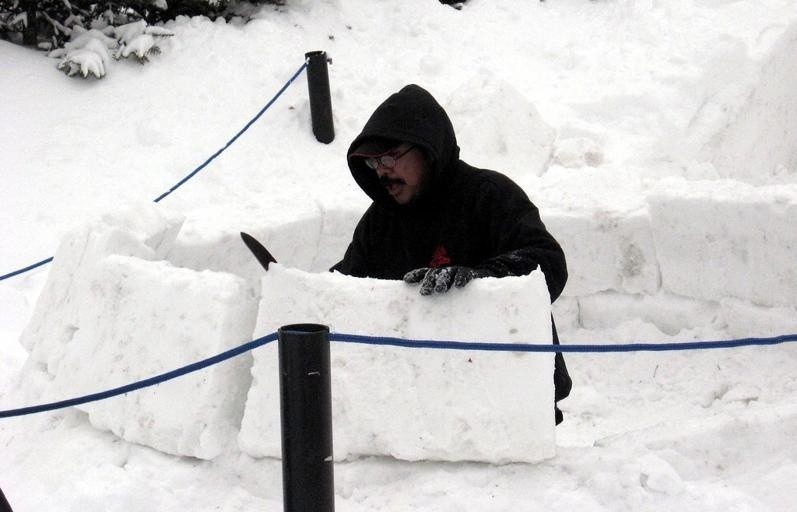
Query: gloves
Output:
[[404, 265, 476, 295]]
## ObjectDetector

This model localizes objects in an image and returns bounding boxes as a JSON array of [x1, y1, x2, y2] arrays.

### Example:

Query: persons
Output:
[[327, 83, 573, 428]]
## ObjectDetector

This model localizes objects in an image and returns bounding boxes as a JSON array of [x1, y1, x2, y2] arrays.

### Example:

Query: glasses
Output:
[[365, 146, 416, 169]]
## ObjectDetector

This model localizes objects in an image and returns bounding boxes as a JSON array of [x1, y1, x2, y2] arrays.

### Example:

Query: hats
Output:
[[348, 132, 402, 157]]
